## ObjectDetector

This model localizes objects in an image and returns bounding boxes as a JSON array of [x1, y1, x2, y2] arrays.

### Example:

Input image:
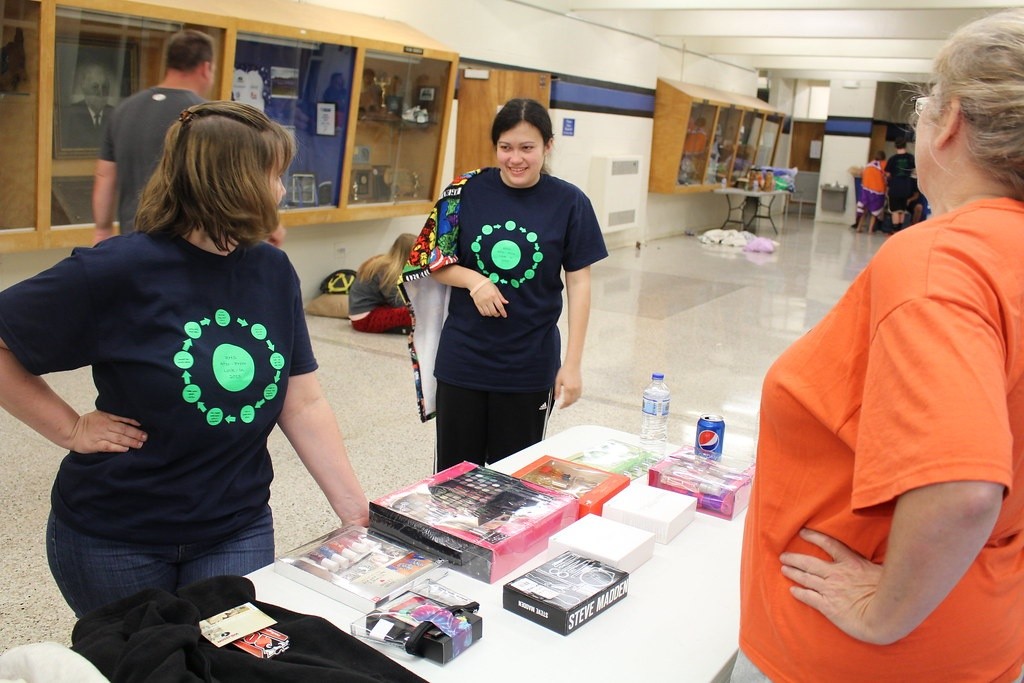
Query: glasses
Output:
[[915, 97, 975, 123], [86, 82, 109, 90]]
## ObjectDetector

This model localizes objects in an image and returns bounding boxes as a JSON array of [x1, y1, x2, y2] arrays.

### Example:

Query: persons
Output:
[[92, 30, 219, 247], [399, 99, 610, 475], [348, 233, 418, 333], [729, 7, 1024, 682], [852, 136, 925, 234], [0, 100, 374, 620], [324, 69, 434, 120], [61, 65, 115, 149]]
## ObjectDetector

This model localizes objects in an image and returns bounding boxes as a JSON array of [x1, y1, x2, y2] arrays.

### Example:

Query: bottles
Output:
[[747, 168, 762, 192], [763, 168, 774, 191], [722, 178, 727, 189], [638, 373, 671, 457]]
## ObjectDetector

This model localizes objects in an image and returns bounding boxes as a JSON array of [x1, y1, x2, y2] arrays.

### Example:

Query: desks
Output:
[[712, 188, 791, 235], [243, 425, 754, 683]]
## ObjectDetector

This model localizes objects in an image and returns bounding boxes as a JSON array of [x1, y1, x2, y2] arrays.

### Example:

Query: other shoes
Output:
[[382, 325, 411, 335]]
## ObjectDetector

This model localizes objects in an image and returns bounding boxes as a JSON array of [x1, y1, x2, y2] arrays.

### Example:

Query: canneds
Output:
[[695, 414, 725, 462]]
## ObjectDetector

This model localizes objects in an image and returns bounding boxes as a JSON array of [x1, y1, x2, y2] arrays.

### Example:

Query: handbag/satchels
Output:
[[320, 269, 356, 294]]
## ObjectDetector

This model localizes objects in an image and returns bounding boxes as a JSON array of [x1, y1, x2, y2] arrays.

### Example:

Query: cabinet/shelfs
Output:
[[0, 0, 460, 256], [650, 77, 786, 193]]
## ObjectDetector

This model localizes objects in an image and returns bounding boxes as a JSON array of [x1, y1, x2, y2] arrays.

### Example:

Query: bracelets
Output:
[[469, 277, 490, 296]]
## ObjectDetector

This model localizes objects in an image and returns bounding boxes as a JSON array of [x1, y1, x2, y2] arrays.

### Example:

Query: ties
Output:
[[93, 112, 101, 127]]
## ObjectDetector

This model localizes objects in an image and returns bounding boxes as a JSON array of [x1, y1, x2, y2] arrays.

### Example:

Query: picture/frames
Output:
[[53, 35, 139, 159]]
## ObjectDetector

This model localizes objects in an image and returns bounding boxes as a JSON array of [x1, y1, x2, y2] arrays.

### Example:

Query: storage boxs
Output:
[[649, 445, 755, 520], [602, 483, 698, 545], [368, 461, 580, 585], [275, 525, 449, 614], [510, 456, 631, 519], [548, 513, 655, 574], [503, 550, 630, 636]]
